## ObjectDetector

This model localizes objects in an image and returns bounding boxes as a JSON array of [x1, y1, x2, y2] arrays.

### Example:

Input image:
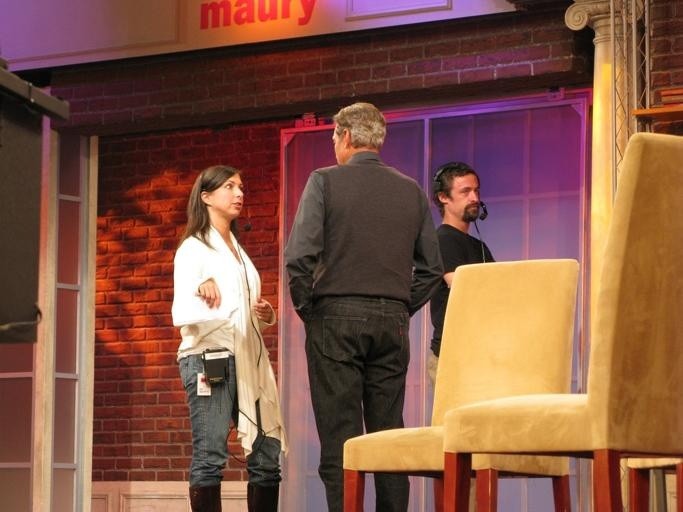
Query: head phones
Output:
[[431, 163, 458, 192]]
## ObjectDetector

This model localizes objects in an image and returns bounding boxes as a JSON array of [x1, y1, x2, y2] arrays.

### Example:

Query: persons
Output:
[[424, 160, 497, 386], [280, 103, 446, 512], [170, 166, 289, 512]]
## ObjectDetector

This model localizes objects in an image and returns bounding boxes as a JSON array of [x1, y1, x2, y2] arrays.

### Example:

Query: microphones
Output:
[[479, 201, 488, 219]]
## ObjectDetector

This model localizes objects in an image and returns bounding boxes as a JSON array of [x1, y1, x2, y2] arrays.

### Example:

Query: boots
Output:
[[247, 482, 279, 512], [189, 484, 222, 512]]
